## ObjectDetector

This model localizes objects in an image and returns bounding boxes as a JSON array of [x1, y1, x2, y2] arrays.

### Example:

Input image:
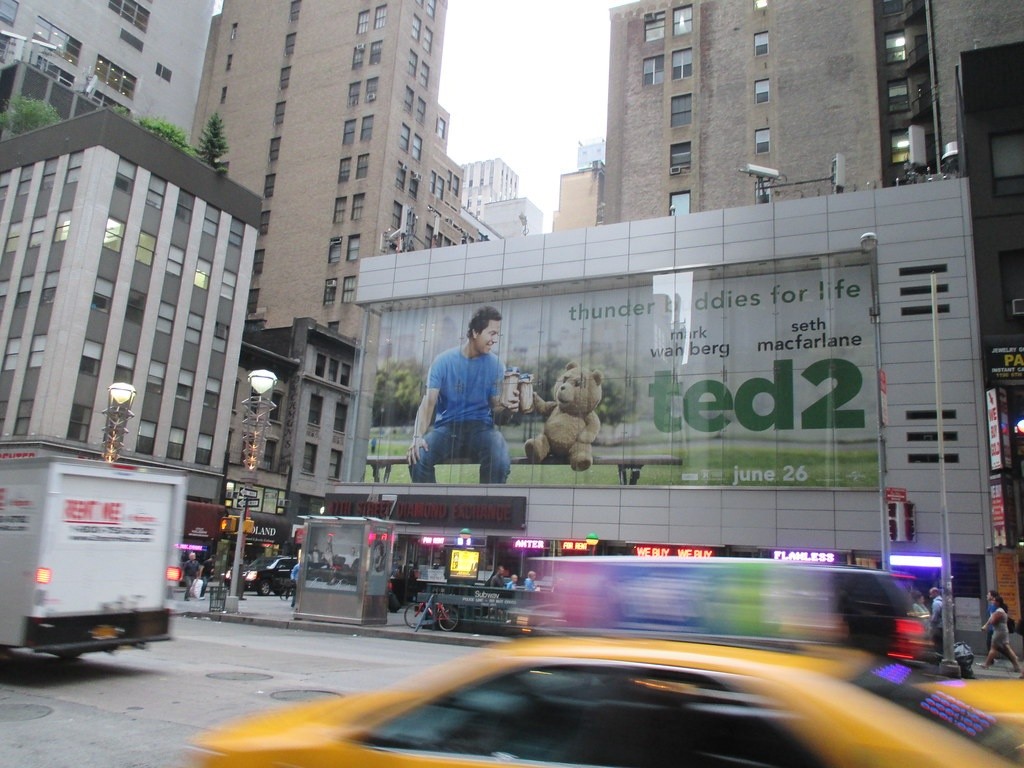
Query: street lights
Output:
[[220, 368, 280, 612], [855, 233, 892, 572], [97, 381, 137, 462]]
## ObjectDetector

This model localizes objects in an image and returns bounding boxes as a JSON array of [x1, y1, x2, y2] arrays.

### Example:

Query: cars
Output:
[[194, 633, 1024, 768]]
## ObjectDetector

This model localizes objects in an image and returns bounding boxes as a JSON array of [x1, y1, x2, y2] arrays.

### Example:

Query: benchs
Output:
[[363, 455, 683, 486]]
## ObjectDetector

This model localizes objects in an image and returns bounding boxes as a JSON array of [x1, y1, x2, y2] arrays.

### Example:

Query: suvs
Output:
[[225, 553, 297, 598]]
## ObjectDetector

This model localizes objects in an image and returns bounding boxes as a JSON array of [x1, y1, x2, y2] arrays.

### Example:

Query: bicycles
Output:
[[403, 592, 460, 633]]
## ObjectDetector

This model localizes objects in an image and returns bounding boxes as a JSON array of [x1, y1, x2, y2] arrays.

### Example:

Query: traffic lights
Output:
[[219, 517, 236, 532]]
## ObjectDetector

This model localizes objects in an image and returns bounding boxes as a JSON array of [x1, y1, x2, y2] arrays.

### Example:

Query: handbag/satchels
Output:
[[189, 578, 203, 599], [1007, 616, 1015, 633]]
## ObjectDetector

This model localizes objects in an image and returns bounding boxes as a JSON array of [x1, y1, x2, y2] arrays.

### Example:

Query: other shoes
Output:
[[976, 661, 990, 670], [199, 596, 205, 600], [184, 598, 189, 600]]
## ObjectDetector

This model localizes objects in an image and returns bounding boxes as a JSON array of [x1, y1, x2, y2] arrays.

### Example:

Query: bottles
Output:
[[519, 373, 534, 413], [501, 367, 519, 408], [536, 585, 540, 591]]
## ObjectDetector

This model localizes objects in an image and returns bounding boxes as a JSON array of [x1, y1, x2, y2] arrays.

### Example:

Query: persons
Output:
[[490, 565, 506, 587], [181, 552, 199, 601], [199, 555, 217, 600], [405, 304, 520, 484], [506, 574, 518, 590], [974, 589, 1024, 679], [290, 563, 301, 607], [388, 560, 414, 613], [309, 544, 322, 563], [371, 437, 376, 452], [524, 570, 537, 592], [912, 584, 943, 654]]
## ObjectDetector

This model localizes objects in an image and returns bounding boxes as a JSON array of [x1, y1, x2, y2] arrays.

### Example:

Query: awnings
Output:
[[221, 508, 291, 545]]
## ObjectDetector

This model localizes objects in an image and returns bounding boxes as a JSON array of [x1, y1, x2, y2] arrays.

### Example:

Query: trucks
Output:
[[0, 456, 189, 662]]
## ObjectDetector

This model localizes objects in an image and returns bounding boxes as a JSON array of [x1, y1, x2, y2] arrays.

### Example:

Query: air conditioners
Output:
[[669, 166, 680, 175], [412, 173, 422, 182], [367, 93, 376, 101], [1007, 299, 1024, 319], [331, 237, 341, 244], [644, 12, 655, 22], [327, 279, 336, 288], [356, 43, 364, 51], [401, 164, 408, 172]]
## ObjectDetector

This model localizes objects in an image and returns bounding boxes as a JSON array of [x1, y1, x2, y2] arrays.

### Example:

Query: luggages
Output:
[[388, 592, 401, 613]]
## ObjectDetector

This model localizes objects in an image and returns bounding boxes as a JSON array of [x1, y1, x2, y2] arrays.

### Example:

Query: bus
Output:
[[526, 554, 935, 676]]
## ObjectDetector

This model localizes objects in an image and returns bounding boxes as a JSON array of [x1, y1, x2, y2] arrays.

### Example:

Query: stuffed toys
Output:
[[523, 361, 603, 472]]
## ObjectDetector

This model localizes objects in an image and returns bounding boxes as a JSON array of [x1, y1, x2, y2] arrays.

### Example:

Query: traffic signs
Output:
[[239, 486, 258, 498], [236, 497, 259, 509]]
